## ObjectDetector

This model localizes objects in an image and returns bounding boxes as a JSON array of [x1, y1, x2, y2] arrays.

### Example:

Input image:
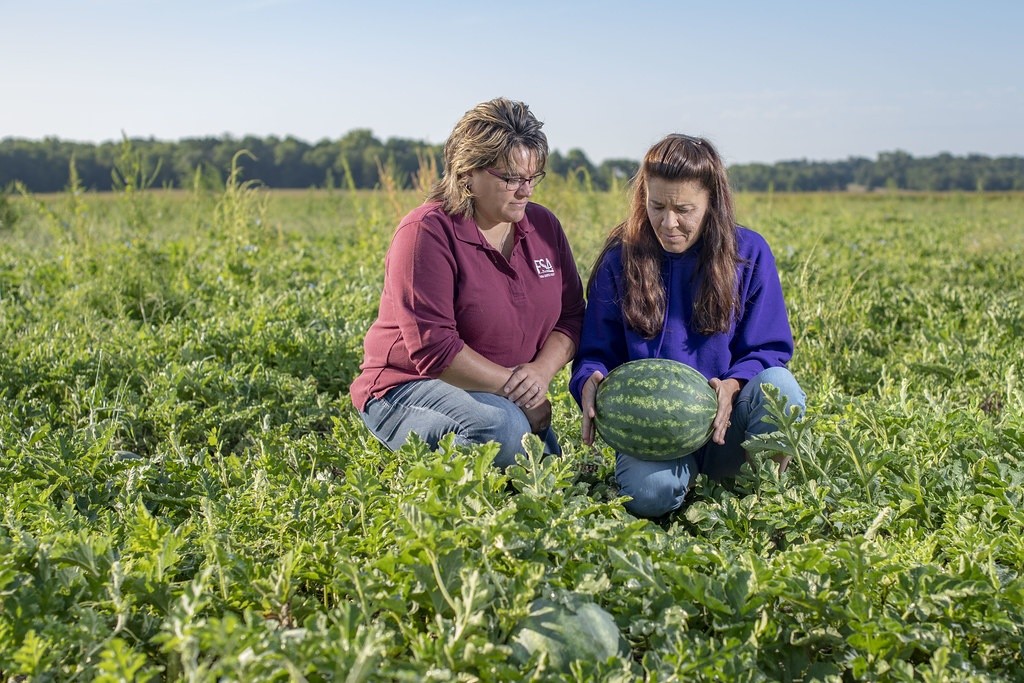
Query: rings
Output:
[[536, 383, 541, 390], [726, 421, 731, 427]]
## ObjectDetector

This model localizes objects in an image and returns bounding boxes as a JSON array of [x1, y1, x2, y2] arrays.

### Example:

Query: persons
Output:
[[350, 99, 585, 494], [568, 133, 807, 531]]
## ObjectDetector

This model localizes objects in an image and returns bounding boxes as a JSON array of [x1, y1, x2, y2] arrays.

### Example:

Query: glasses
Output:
[[481, 167, 546, 190]]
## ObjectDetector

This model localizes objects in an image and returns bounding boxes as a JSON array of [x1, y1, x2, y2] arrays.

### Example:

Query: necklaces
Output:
[[477, 225, 510, 251]]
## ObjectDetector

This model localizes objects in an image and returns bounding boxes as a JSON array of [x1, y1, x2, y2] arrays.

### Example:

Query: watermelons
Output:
[[505, 595, 634, 669], [595, 358, 719, 460]]
